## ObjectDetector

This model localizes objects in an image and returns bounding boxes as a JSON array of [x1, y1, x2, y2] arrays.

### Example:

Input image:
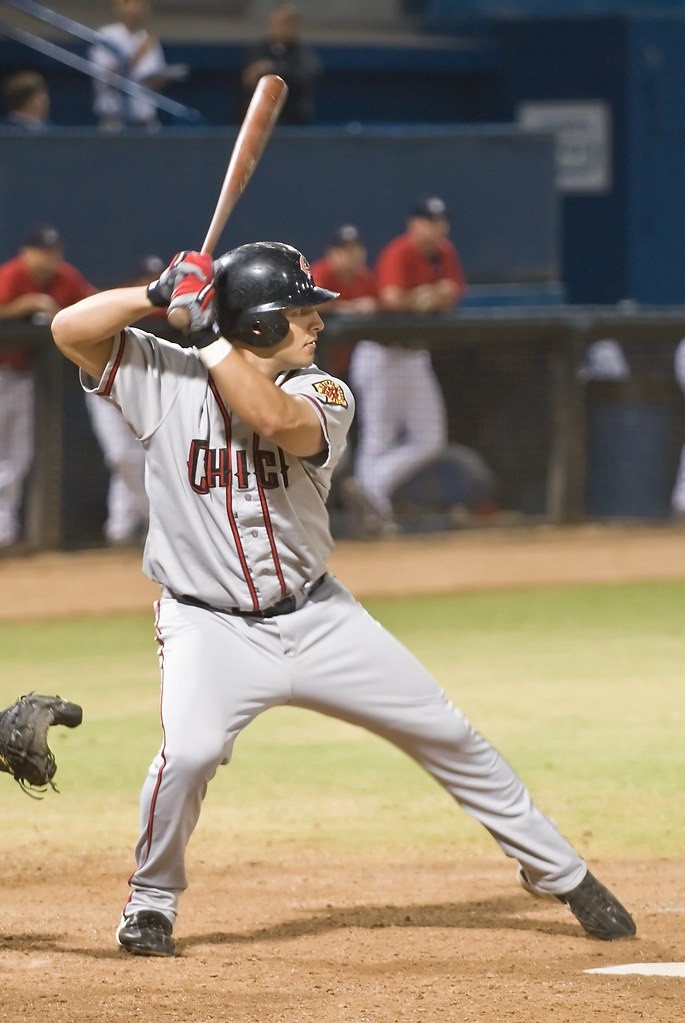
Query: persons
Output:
[[49, 239, 639, 957], [0, 227, 165, 557], [244, 5, 316, 125], [307, 195, 467, 542], [0, 71, 66, 139], [86, 1, 168, 130]]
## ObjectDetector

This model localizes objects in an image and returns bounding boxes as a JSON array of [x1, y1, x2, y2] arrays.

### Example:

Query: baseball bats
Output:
[[166, 75, 287, 327]]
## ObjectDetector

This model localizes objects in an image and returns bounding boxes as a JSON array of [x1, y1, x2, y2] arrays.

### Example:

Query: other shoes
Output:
[[338, 478, 404, 541]]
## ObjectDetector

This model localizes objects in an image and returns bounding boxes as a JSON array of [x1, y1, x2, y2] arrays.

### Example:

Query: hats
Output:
[[330, 225, 363, 247], [21, 227, 65, 249], [412, 197, 452, 220]]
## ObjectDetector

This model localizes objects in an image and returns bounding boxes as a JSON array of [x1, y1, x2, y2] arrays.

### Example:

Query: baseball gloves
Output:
[[0, 689, 83, 801]]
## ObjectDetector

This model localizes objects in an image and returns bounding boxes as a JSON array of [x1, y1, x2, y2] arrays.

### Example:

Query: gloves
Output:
[[147, 249, 225, 349]]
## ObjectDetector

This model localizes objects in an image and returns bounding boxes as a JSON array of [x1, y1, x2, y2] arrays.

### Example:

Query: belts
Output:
[[175, 572, 326, 618]]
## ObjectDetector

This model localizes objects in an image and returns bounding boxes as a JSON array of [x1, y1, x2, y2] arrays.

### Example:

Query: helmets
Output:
[[211, 242, 342, 348]]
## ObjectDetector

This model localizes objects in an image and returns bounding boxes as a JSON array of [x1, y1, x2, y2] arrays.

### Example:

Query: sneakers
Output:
[[115, 910, 175, 957], [517, 865, 636, 941]]
